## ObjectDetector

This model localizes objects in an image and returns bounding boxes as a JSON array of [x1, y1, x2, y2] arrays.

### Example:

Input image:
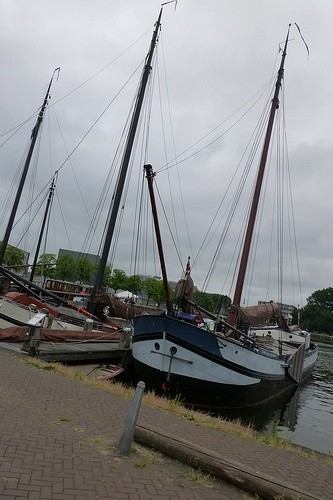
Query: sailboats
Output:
[[0, 9, 258, 364], [115, 22, 319, 423]]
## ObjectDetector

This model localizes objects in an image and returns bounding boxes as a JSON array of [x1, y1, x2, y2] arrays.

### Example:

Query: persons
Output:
[[116, 297, 137, 306]]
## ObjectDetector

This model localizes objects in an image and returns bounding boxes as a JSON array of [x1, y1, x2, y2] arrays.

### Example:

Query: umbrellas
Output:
[[114, 291, 139, 298]]
